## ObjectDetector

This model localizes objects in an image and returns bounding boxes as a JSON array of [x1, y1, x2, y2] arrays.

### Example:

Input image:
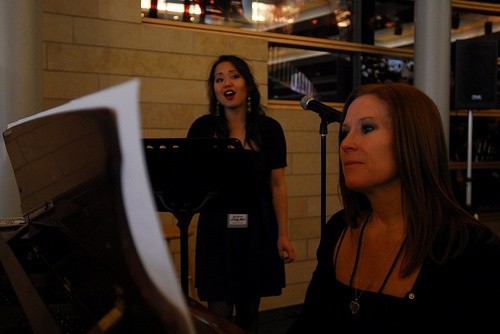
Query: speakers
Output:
[[450, 40, 500, 111]]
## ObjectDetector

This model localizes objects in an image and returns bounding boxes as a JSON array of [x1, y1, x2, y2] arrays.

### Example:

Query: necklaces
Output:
[[349, 214, 406, 314]]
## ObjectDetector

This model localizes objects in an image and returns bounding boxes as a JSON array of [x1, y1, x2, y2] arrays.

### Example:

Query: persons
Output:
[[296, 84, 499, 334], [184, 55, 296, 334]]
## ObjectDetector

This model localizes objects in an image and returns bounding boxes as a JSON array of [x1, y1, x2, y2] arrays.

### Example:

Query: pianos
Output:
[[0, 108, 250, 334]]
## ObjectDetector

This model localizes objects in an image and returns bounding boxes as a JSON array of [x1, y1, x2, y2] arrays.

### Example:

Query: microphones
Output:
[[300, 96, 343, 123]]
